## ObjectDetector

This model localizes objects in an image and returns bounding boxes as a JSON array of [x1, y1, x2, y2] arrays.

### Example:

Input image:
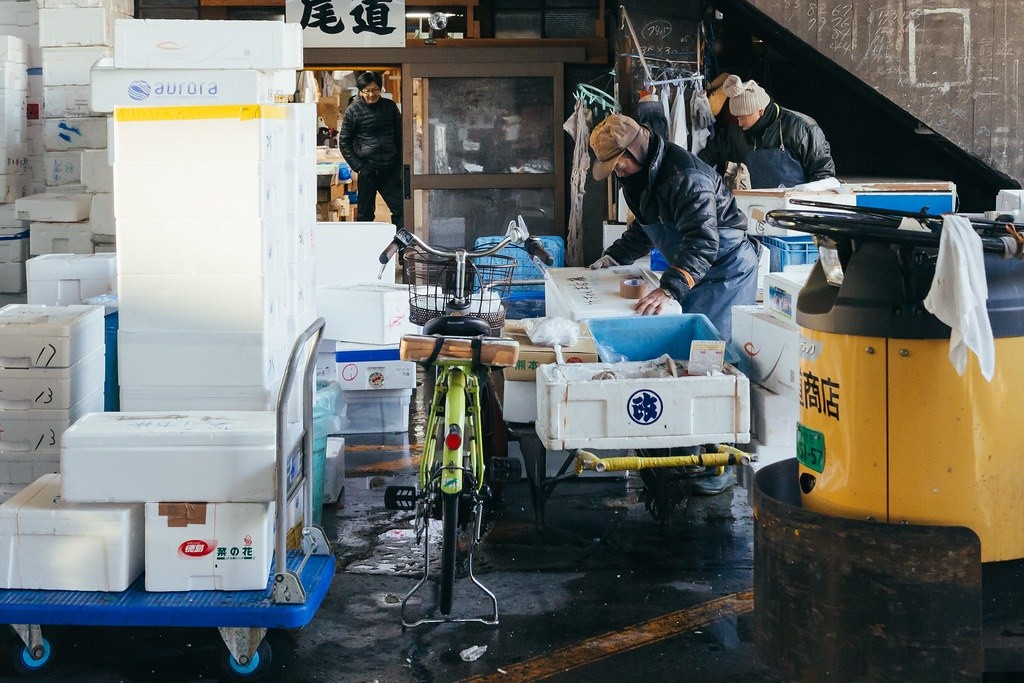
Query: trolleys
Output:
[[0, 312, 339, 683]]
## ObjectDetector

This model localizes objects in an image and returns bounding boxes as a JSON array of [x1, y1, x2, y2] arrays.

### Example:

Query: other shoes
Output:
[[690, 467, 707, 473], [694, 464, 737, 495]]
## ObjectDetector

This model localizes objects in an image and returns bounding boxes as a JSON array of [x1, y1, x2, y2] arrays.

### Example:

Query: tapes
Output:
[[620, 278, 650, 299]]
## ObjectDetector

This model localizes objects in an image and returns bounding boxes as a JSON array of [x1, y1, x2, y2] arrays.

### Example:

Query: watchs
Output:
[[662, 288, 674, 298]]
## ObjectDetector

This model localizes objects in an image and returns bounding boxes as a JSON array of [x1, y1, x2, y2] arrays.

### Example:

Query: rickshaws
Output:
[[374, 214, 761, 634]]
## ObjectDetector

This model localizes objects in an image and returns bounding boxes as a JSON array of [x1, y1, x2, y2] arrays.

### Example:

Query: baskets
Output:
[[404, 247, 516, 332]]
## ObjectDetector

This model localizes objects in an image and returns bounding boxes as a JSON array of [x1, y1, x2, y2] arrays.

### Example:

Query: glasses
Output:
[[361, 88, 380, 94]]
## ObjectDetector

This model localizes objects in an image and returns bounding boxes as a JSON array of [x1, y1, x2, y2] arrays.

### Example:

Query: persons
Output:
[[698, 74, 835, 188], [589, 113, 759, 493], [339, 71, 405, 264]]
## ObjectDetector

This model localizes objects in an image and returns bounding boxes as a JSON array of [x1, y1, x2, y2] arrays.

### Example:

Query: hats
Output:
[[723, 75, 771, 116], [589, 113, 649, 180]]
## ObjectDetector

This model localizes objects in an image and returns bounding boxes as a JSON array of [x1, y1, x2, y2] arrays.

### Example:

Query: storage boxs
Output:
[[0, 0, 424, 592], [471, 187, 856, 481]]
